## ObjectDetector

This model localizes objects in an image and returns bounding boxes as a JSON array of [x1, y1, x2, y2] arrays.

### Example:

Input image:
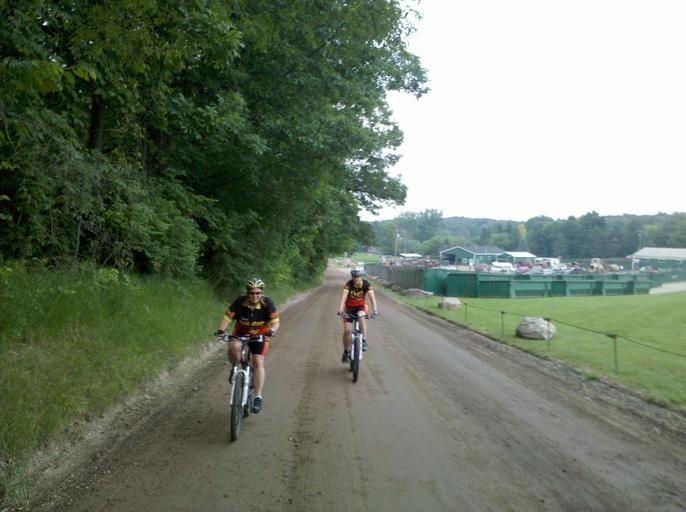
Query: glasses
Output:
[[247, 291, 263, 295]]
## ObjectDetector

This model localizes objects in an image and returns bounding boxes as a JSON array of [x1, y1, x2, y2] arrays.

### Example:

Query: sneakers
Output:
[[342, 349, 350, 362], [361, 336, 367, 351], [252, 396, 262, 413], [229, 368, 237, 383]]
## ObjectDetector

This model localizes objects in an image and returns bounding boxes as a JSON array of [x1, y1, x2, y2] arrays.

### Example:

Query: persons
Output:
[[218, 278, 281, 413], [338, 269, 378, 362]]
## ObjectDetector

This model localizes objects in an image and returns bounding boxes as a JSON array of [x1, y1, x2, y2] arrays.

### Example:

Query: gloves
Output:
[[216, 329, 224, 334], [265, 329, 273, 337]]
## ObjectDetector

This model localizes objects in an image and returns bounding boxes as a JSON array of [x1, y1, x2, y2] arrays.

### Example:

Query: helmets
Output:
[[351, 267, 364, 275], [246, 277, 265, 289]]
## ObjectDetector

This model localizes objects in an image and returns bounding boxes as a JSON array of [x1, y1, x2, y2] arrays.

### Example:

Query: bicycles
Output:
[[337, 312, 372, 383], [212, 332, 278, 440]]
